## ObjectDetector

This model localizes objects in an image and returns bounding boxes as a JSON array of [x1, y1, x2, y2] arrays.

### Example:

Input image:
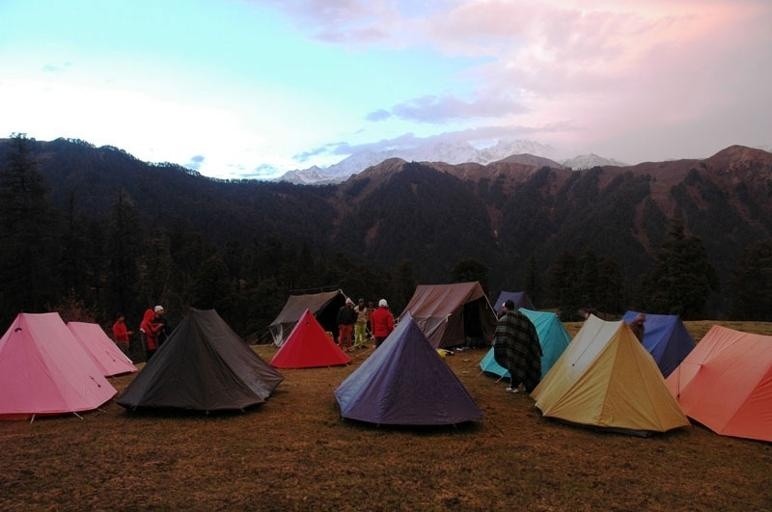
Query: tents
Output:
[[1, 313, 137, 413], [116, 309, 282, 413], [269, 289, 369, 344], [271, 308, 351, 368], [479, 307, 571, 384], [335, 311, 482, 428], [398, 282, 535, 349], [533, 310, 772, 442]]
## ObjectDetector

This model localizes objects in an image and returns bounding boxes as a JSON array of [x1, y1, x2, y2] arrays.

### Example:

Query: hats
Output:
[[379, 299, 387, 307], [154, 306, 163, 312]]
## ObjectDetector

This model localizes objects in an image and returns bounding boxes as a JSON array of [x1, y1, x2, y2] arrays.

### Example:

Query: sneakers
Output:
[[506, 388, 518, 392]]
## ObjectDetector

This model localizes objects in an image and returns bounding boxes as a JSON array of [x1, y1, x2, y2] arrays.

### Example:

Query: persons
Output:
[[494, 300, 544, 393], [112, 304, 176, 359], [338, 296, 394, 352]]
